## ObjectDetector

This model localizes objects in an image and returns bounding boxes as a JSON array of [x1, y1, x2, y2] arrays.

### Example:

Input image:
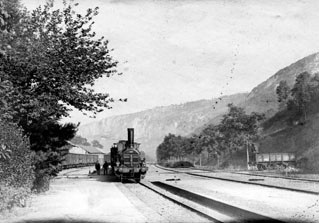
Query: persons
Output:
[[95, 159, 101, 175], [103, 160, 108, 175]]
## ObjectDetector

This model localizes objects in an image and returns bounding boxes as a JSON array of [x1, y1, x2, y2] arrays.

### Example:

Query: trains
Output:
[[57, 153, 98, 171], [104, 128, 148, 183]]
